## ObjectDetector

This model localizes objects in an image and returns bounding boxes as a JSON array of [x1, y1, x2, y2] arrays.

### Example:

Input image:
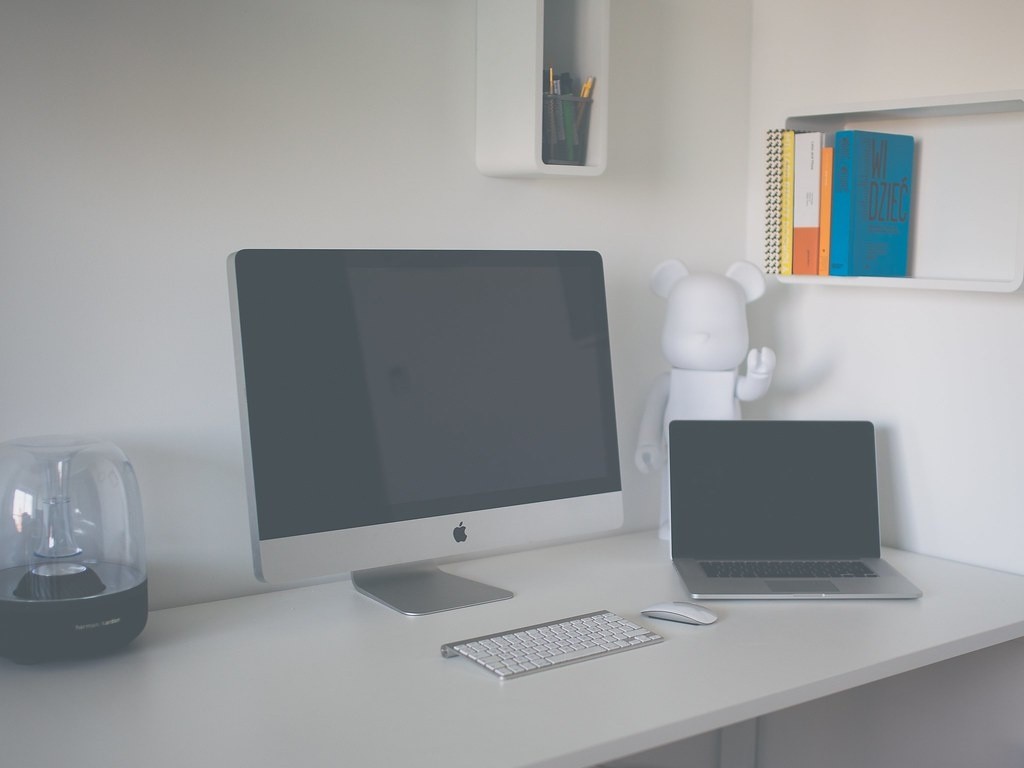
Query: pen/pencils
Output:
[[549, 64, 554, 140], [578, 77, 593, 126]]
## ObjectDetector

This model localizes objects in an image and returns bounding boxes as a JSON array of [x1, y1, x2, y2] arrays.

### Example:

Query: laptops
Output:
[[669, 418, 923, 602]]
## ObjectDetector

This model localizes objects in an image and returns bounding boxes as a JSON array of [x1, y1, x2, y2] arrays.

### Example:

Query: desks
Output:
[[0, 527, 1023, 767]]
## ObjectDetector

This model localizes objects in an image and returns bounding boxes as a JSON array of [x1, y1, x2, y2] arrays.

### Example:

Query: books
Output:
[[765, 127, 914, 277]]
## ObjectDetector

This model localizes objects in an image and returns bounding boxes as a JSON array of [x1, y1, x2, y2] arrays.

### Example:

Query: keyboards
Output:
[[439, 610, 666, 680]]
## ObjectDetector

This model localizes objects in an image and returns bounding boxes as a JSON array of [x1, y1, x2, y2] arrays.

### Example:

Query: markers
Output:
[[543, 70, 581, 162]]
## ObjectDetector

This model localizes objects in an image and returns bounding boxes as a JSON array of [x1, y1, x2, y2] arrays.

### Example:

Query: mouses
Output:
[[640, 603, 718, 625]]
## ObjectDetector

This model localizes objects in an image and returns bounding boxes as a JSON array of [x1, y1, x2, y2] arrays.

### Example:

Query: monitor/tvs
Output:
[[226, 244, 628, 620]]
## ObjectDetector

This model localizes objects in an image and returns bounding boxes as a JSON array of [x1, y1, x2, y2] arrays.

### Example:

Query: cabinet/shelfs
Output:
[[748, 86, 1024, 293]]
